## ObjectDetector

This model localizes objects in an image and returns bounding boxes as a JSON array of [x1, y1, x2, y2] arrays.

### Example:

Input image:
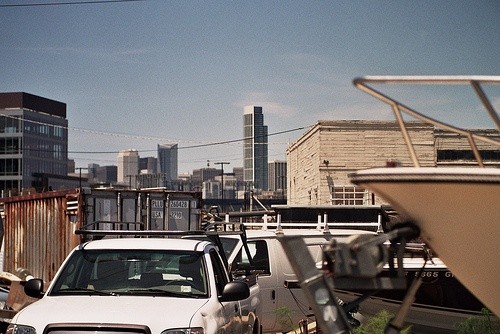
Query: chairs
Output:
[[167, 256, 202, 287], [86, 260, 148, 291]]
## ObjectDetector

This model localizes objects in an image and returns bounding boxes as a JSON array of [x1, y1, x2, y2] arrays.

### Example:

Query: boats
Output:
[[312, 65, 499, 334]]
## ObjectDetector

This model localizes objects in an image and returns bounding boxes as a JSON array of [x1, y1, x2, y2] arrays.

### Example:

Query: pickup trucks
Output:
[[5, 225, 262, 334]]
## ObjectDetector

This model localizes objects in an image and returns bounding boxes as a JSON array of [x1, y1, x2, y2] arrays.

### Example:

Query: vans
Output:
[[181, 228, 382, 332]]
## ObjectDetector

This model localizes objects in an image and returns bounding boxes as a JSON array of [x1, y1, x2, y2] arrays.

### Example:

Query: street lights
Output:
[[214, 162, 230, 213]]
